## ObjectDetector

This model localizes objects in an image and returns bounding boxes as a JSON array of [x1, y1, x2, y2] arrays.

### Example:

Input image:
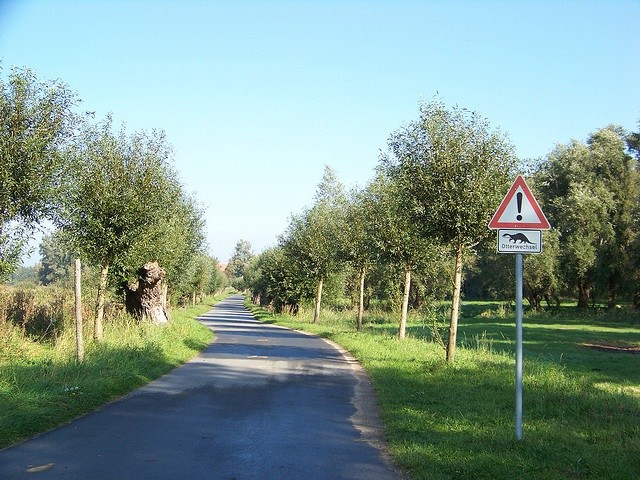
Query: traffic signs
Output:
[[488, 174, 551, 231], [496, 229, 542, 254]]
